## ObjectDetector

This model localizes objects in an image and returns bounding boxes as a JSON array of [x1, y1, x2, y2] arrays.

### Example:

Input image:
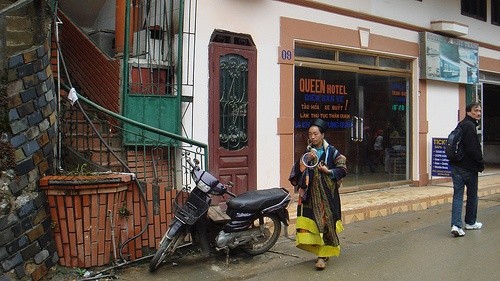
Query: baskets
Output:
[[171, 187, 210, 225]]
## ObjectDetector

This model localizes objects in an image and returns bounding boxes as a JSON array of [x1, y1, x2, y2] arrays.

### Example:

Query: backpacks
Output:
[[445, 118, 477, 165]]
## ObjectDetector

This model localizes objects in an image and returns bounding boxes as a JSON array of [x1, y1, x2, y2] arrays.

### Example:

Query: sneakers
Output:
[[450, 225, 466, 236], [465, 220, 482, 230]]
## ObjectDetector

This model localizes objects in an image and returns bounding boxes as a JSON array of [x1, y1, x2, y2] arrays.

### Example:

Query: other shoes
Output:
[[314, 256, 329, 268]]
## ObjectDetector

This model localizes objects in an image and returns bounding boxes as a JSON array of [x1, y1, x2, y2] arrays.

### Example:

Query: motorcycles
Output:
[[149, 158, 294, 273]]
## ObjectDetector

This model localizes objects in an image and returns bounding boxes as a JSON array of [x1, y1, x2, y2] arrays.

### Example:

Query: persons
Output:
[[445, 102, 486, 237], [287, 118, 348, 269]]
